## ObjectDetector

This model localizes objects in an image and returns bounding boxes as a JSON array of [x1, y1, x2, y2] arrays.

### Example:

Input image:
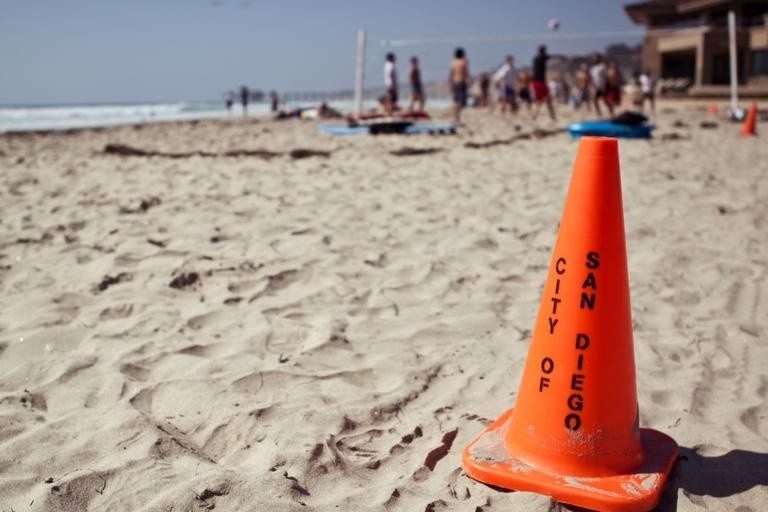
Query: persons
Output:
[[448, 47, 469, 124], [341, 94, 389, 118], [477, 72, 490, 111], [491, 54, 520, 112], [226, 90, 234, 111], [383, 52, 398, 115], [517, 55, 658, 120], [405, 57, 426, 112], [269, 90, 281, 111], [240, 85, 249, 111], [525, 42, 560, 123]]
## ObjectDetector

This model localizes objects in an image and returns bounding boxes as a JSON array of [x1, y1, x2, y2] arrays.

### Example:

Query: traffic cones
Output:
[[462, 133, 681, 510], [738, 102, 760, 137]]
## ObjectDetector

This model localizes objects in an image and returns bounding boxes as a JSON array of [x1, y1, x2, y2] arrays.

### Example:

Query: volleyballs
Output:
[[547, 18, 559, 31]]
